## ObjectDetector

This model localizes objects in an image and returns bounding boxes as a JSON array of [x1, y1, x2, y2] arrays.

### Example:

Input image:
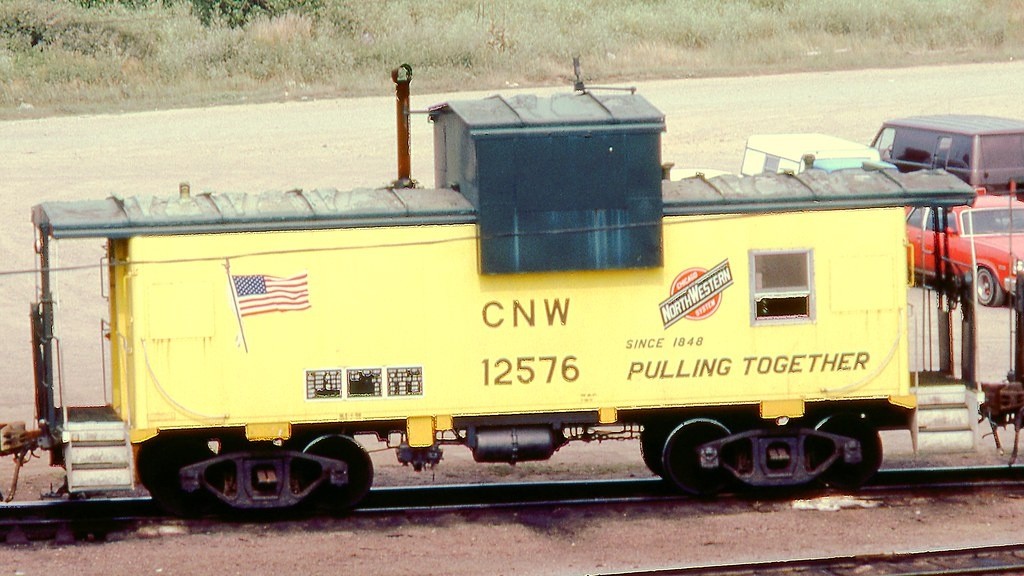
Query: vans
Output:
[[869, 115, 1024, 200], [742, 130, 898, 180]]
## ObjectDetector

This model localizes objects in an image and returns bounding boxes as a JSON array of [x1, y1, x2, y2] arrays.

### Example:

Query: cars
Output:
[[904, 191, 1024, 308]]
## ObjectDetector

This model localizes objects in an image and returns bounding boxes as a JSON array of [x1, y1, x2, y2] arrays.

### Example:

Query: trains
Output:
[[0, 51, 1024, 520]]
